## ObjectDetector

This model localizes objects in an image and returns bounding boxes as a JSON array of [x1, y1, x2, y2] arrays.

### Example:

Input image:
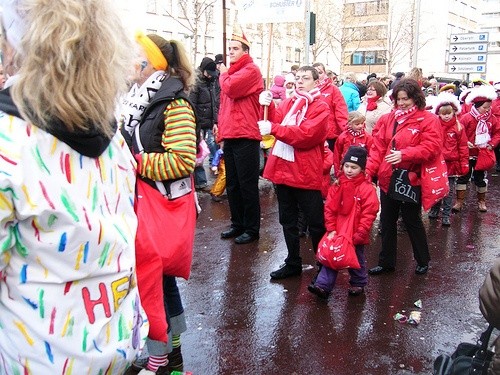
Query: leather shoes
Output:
[[235, 233, 259, 243], [369, 265, 394, 274], [415, 264, 428, 274], [221, 228, 243, 238]]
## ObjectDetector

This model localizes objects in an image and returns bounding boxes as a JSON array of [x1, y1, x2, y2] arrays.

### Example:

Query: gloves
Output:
[[257, 120, 272, 136], [259, 90, 273, 107], [459, 159, 470, 176]]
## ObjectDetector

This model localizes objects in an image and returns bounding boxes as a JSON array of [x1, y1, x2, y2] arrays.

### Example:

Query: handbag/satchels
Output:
[[136, 178, 197, 281], [386, 170, 421, 205], [474, 146, 496, 170], [419, 150, 450, 210], [315, 219, 362, 269]]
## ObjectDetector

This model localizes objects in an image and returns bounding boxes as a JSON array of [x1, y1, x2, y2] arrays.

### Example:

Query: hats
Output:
[[342, 146, 367, 169], [433, 82, 500, 115], [231, 10, 250, 47], [206, 63, 216, 76]]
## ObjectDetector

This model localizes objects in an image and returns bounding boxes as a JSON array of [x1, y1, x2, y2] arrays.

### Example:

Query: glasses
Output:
[[366, 88, 376, 91]]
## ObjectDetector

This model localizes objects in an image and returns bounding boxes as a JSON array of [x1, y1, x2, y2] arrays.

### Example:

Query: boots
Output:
[[476, 185, 487, 211], [452, 184, 467, 211]]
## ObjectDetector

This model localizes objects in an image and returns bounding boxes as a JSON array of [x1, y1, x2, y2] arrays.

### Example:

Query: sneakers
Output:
[[271, 265, 303, 279]]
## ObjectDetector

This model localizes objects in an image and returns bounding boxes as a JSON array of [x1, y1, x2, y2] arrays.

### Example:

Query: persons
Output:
[[1, 61, 19, 90], [0, 0, 155, 375], [189, 26, 500, 244], [113, 29, 200, 375], [477, 256, 500, 375], [365, 77, 443, 275], [308, 146, 377, 299], [256, 66, 330, 279]]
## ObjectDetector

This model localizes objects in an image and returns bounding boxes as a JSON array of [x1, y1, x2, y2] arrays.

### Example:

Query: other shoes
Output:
[[429, 210, 440, 218], [212, 194, 222, 201], [443, 217, 450, 225], [309, 284, 329, 297], [133, 353, 183, 369], [349, 286, 363, 295]]
